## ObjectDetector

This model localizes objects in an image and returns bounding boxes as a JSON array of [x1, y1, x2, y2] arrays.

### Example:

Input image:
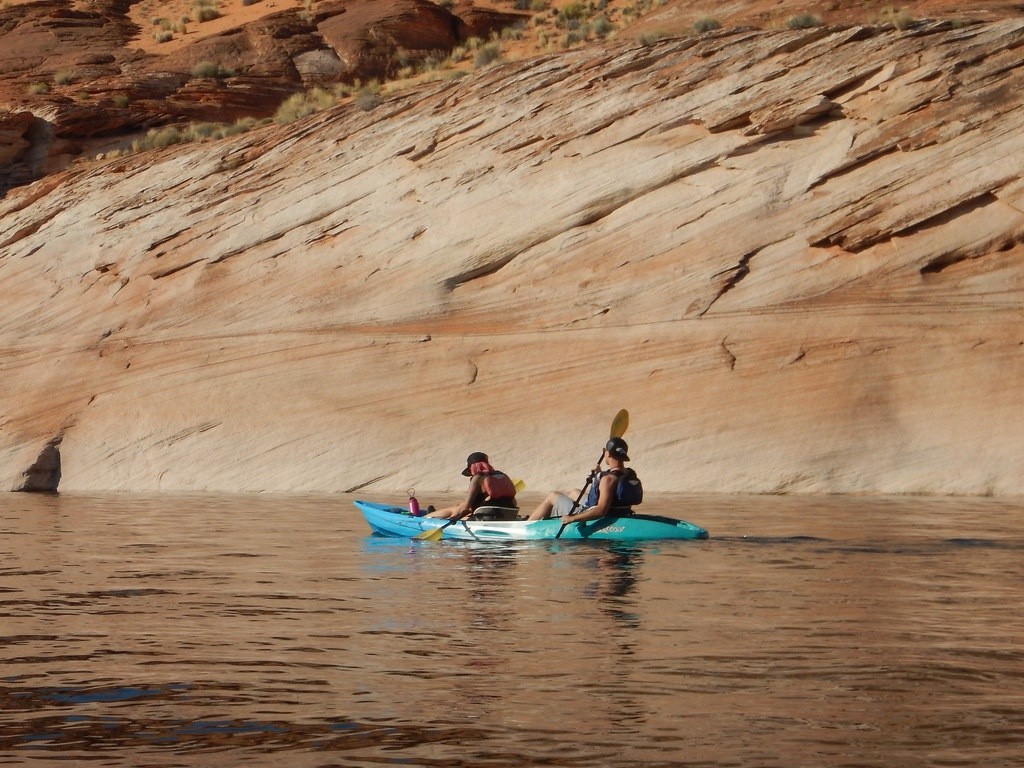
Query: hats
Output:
[[461, 452, 488, 477], [606, 438, 631, 462]]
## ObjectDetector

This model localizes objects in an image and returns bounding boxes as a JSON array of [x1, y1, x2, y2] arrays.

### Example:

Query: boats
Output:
[[354, 499, 710, 542]]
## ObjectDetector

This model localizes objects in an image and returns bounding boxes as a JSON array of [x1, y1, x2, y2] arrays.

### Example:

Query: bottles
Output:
[[407, 488, 419, 516]]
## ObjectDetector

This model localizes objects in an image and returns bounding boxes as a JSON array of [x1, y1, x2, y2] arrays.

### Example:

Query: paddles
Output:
[[418, 479, 525, 541], [554, 408, 630, 540]]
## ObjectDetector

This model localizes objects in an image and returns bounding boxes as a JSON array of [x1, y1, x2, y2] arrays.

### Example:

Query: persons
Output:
[[422, 452, 517, 521], [525, 437, 638, 525]]
